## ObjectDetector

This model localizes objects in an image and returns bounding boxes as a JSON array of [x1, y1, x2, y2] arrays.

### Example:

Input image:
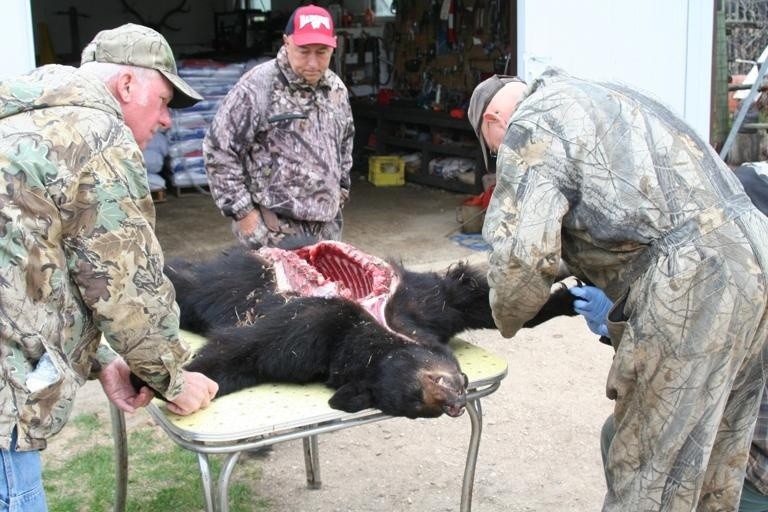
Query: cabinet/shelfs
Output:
[[329, 26, 393, 96], [353, 98, 517, 196]]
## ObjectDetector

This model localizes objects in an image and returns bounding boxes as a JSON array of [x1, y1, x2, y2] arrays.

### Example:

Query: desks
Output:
[[100, 251, 510, 512]]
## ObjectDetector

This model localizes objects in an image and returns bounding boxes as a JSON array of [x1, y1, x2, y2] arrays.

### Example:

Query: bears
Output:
[[129, 230, 584, 419]]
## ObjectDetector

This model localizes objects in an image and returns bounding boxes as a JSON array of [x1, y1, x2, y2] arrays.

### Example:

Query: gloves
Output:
[[570, 284, 615, 337]]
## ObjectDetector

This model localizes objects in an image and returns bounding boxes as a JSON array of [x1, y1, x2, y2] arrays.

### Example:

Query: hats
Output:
[[284, 4, 337, 48], [466, 73, 527, 174], [80, 23, 204, 109]]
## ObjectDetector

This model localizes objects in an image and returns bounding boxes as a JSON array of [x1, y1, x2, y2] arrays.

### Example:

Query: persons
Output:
[[0, 19, 224, 512], [566, 160, 768, 511], [460, 69, 768, 511], [202, 4, 356, 455]]
